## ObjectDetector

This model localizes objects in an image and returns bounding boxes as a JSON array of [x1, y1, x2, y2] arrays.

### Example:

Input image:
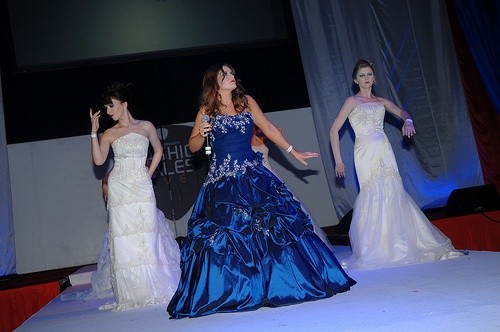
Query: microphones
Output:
[[201, 115, 212, 162]]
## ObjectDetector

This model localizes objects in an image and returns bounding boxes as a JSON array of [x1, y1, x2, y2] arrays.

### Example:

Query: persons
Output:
[[251, 125, 334, 252], [59, 88, 181, 312], [330, 58, 463, 269], [167, 63, 357, 319]]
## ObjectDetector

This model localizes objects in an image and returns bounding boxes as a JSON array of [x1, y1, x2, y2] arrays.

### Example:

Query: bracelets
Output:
[[286, 145, 293, 153], [91, 135, 96, 137]]
